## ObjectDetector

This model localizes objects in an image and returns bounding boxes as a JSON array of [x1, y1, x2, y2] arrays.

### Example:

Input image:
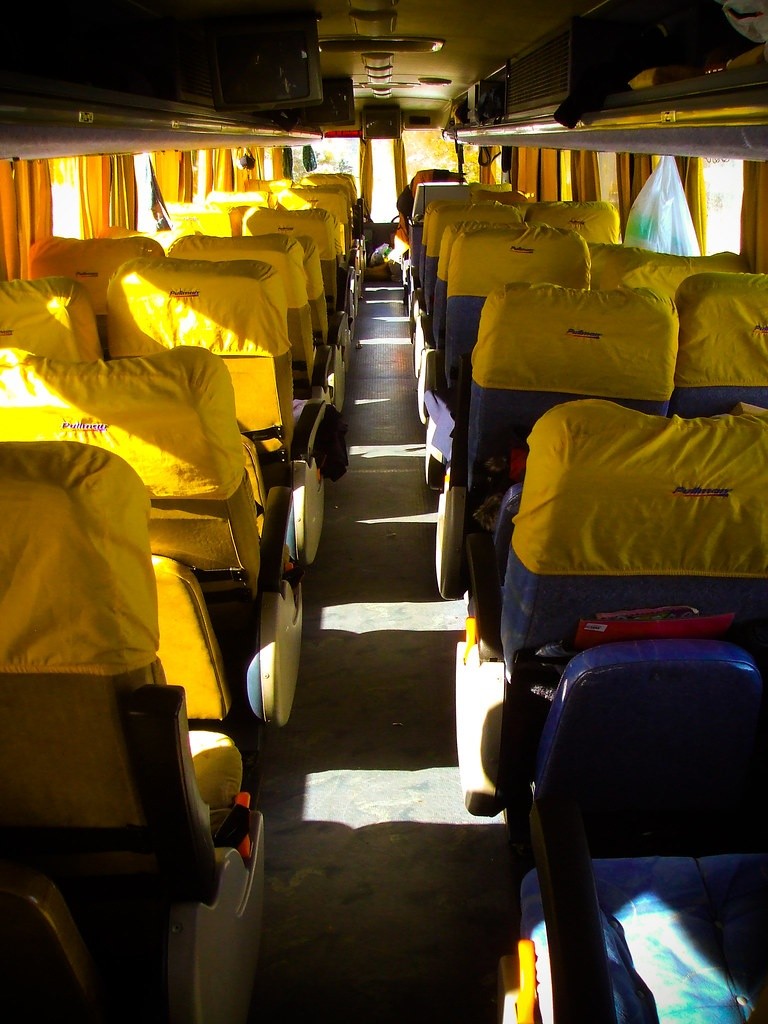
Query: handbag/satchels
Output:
[[397, 184, 413, 216], [502, 606, 737, 776], [623, 155, 700, 257]]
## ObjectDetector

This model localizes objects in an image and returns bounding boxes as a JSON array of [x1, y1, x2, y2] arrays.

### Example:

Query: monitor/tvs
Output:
[[362, 105, 401, 140], [300, 78, 356, 127], [203, 10, 324, 114]]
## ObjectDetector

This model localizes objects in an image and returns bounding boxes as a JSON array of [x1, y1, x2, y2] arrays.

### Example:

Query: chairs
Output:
[[384, 168, 768, 1024], [1, 167, 370, 1023]]
